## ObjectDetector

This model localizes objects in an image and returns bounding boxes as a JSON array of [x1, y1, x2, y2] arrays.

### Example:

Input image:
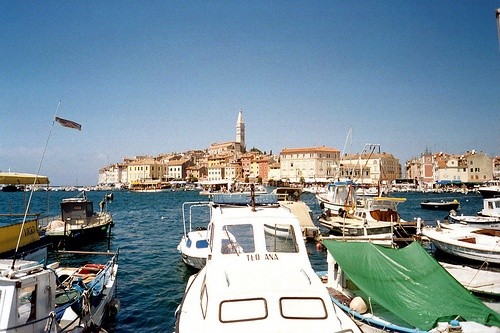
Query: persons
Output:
[[209, 185, 244, 193], [26, 284, 38, 323], [99, 200, 105, 213], [448, 209, 457, 224]]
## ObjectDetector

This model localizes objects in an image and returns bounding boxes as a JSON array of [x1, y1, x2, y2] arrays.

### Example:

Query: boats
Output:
[[175, 182, 363, 333], [421, 199, 460, 210], [321, 239, 500, 333], [422, 180, 500, 263], [45, 192, 113, 237], [316, 126, 423, 235], [0, 99, 119, 333]]
